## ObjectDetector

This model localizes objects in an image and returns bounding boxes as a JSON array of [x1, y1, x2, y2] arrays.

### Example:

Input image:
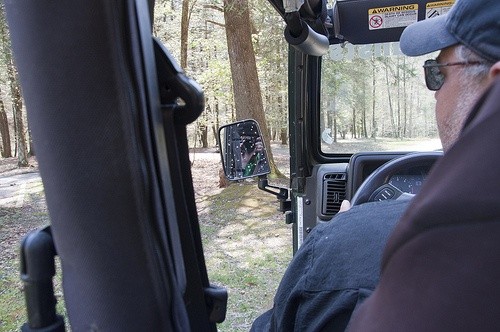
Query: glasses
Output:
[[241, 149, 253, 155], [422, 59, 484, 91]]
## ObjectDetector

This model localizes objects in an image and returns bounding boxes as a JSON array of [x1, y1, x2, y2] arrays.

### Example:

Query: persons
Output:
[[240, 137, 265, 176], [248, 0, 499, 332]]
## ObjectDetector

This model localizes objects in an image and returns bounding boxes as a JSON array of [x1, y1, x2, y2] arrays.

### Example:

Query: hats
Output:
[[240, 139, 255, 152], [399, 0, 500, 63]]
